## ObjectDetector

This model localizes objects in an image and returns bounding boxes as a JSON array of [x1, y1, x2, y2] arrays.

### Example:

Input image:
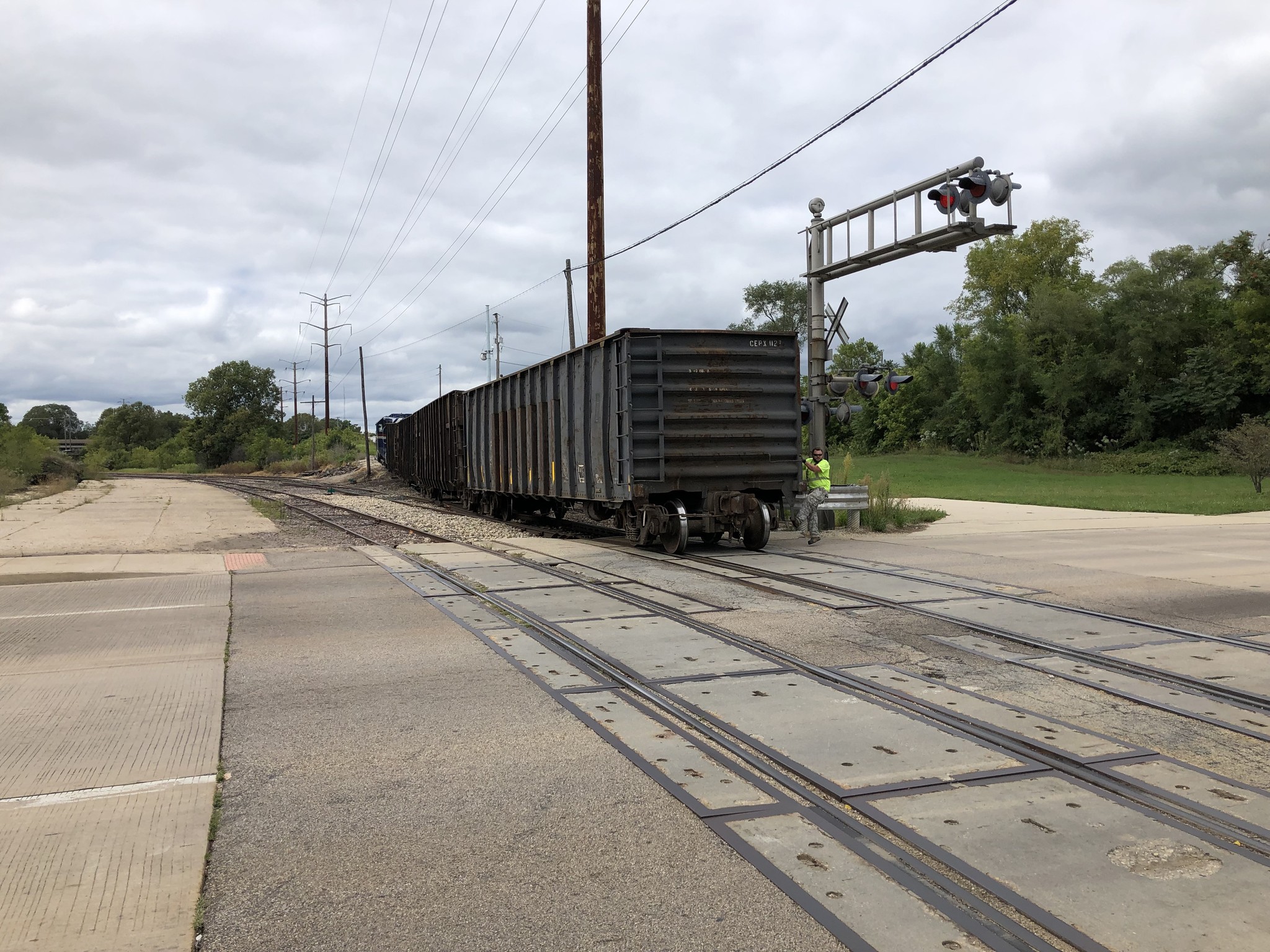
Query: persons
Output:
[[790, 447, 831, 545]]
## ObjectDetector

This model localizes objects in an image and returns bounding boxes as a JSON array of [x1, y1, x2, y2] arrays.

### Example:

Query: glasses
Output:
[[813, 453, 823, 456]]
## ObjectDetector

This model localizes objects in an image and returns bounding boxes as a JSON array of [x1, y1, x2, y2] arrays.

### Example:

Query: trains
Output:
[[375, 327, 806, 556]]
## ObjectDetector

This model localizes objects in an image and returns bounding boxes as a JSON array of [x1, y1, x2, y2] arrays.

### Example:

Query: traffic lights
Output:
[[927, 183, 961, 215], [884, 371, 914, 395], [958, 168, 993, 204], [836, 402, 863, 425], [824, 403, 837, 428], [852, 369, 883, 395], [991, 174, 1022, 206]]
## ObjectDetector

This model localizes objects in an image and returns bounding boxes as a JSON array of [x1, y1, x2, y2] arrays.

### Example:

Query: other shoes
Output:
[[790, 517, 799, 528], [808, 537, 821, 545]]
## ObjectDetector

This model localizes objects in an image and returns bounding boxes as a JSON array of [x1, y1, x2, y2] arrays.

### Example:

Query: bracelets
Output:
[[803, 459, 806, 464]]
